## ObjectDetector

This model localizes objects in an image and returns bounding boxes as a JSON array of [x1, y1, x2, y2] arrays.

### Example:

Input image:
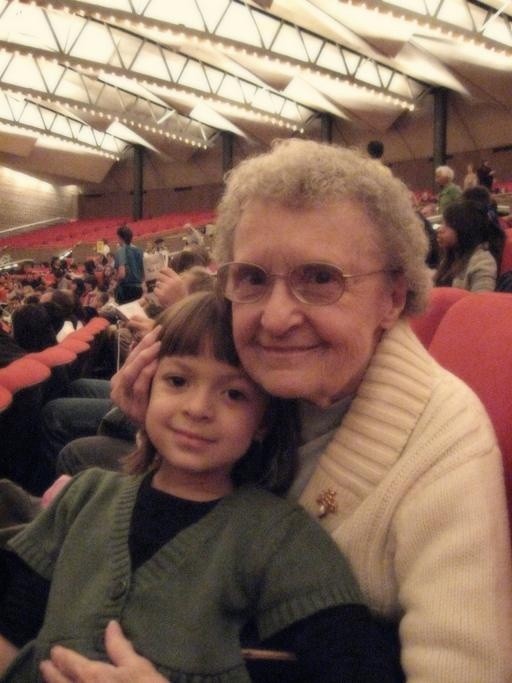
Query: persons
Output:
[[0, 141, 511, 682]]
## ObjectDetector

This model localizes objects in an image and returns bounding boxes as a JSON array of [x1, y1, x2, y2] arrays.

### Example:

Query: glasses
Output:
[[216, 262, 403, 306]]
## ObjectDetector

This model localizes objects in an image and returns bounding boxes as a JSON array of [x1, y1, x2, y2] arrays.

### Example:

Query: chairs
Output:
[[1, 179, 512, 538]]
